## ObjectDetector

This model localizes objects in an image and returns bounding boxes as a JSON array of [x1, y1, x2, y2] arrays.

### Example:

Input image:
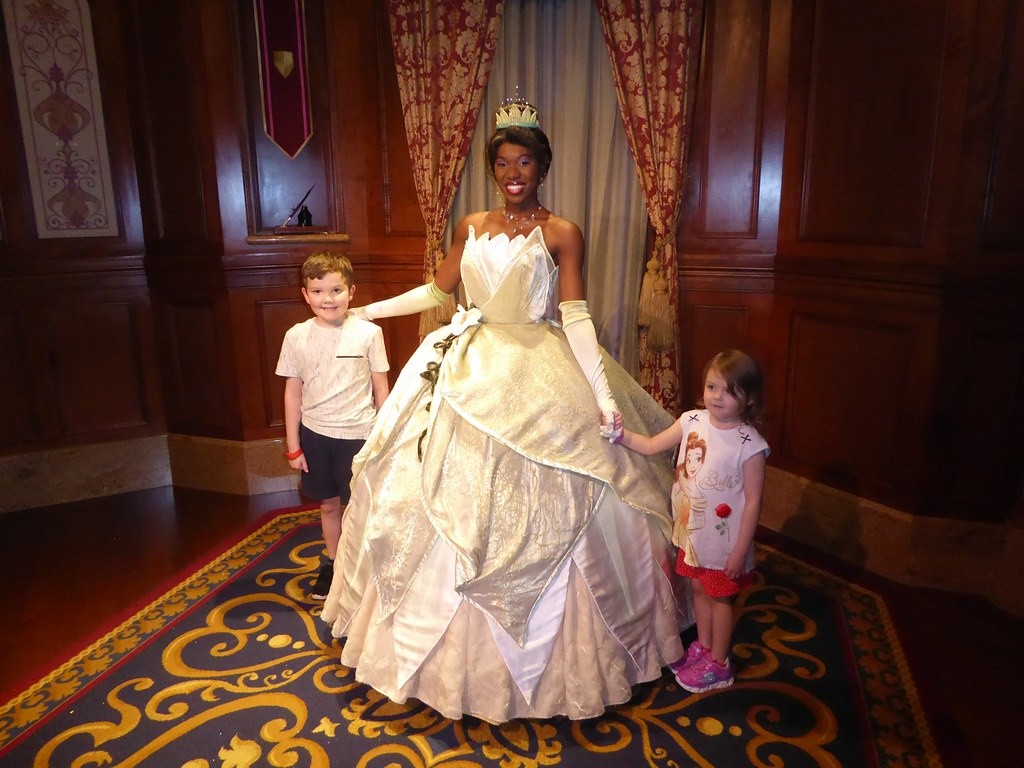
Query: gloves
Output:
[[345, 278, 454, 320], [557, 300, 622, 444]]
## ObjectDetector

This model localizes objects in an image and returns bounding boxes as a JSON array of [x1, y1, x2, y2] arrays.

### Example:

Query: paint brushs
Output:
[[282, 184, 315, 226]]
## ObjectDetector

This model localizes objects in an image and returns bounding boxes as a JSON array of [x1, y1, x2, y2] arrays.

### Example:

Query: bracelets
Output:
[[283, 448, 304, 460], [614, 428, 624, 443]]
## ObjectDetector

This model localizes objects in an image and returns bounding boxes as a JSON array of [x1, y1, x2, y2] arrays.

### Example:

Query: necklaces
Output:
[[501, 201, 541, 234]]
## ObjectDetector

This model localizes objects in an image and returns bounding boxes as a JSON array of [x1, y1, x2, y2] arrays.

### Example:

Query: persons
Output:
[[318, 98, 695, 726], [600, 349, 772, 693], [273, 249, 390, 599]]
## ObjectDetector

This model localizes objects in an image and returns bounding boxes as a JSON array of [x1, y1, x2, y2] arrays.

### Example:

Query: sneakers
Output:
[[667, 641, 711, 674], [676, 653, 734, 693], [311, 564, 334, 600]]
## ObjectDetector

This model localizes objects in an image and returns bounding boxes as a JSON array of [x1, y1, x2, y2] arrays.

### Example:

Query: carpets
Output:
[[1, 500, 972, 767]]
